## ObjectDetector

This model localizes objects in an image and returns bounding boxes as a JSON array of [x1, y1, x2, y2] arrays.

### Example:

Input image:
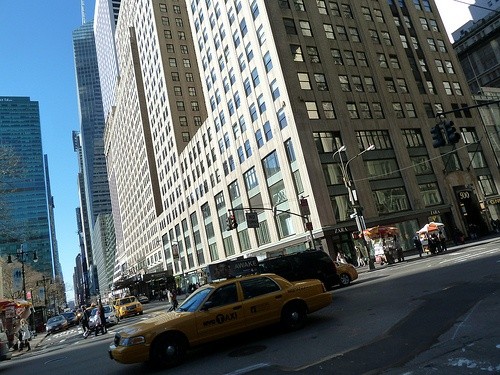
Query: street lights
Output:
[[36, 275, 54, 323], [333, 144, 378, 270], [8, 243, 38, 301]]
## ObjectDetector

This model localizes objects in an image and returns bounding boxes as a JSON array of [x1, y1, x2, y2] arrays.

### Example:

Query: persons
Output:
[[97, 303, 108, 333], [155, 287, 183, 301], [468, 224, 478, 240], [166, 287, 179, 312], [188, 282, 200, 293], [336, 236, 404, 268], [91, 303, 106, 336], [78, 306, 91, 332], [19, 319, 31, 352], [414, 230, 448, 257], [454, 227, 465, 242]]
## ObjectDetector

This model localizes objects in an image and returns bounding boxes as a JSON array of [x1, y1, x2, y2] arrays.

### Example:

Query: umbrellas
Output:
[[416, 222, 444, 235], [0, 298, 32, 313], [352, 226, 399, 238]]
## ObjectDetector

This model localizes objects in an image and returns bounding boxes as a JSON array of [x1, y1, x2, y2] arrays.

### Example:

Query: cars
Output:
[[89, 306, 119, 330], [44, 315, 69, 334], [333, 261, 358, 286], [0, 321, 13, 361], [113, 296, 144, 320], [63, 306, 95, 327], [108, 256, 331, 369], [140, 296, 148, 303]]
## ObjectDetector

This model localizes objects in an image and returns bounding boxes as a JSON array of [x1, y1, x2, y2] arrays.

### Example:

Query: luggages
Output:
[[83, 330, 92, 339]]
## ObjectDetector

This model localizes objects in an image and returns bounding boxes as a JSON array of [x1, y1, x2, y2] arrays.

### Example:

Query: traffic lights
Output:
[[430, 125, 446, 149], [225, 218, 231, 231], [441, 118, 462, 145], [229, 215, 238, 229]]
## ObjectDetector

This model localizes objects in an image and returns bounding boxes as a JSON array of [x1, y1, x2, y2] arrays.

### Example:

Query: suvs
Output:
[[252, 248, 340, 292]]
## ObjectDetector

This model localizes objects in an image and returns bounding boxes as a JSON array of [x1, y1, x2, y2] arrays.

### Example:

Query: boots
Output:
[[18, 343, 24, 352], [27, 342, 31, 352]]
